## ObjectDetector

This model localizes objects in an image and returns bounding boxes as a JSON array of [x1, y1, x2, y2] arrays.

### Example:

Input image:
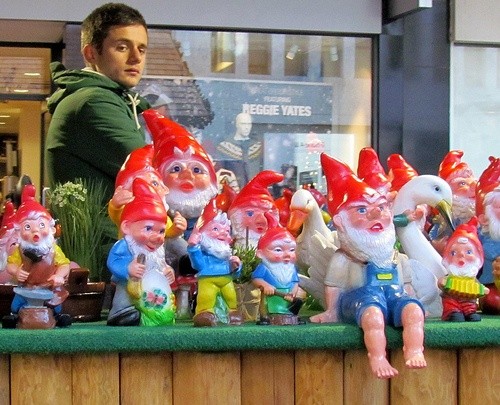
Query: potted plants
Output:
[[231, 246, 262, 321]]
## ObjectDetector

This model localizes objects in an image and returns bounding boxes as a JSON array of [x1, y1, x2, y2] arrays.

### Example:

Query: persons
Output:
[[432, 151, 482, 252], [186, 189, 244, 328], [41, 2, 165, 276], [110, 144, 187, 270], [473, 157, 500, 313], [141, 109, 219, 247], [275, 184, 336, 240], [251, 213, 308, 325], [439, 216, 490, 322], [0, 184, 28, 272], [385, 154, 420, 199], [310, 154, 427, 378], [222, 112, 263, 186], [358, 148, 399, 208], [106, 180, 175, 326], [7, 184, 75, 329], [230, 170, 286, 281]]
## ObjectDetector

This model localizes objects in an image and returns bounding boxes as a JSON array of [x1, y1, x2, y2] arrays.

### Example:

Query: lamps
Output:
[[330, 45, 339, 61], [287, 45, 300, 60]]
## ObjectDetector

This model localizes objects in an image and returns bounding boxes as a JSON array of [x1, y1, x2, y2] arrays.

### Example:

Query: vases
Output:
[[61, 280, 106, 321]]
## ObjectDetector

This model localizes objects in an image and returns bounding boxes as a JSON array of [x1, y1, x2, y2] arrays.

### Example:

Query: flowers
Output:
[[43, 175, 112, 283]]
